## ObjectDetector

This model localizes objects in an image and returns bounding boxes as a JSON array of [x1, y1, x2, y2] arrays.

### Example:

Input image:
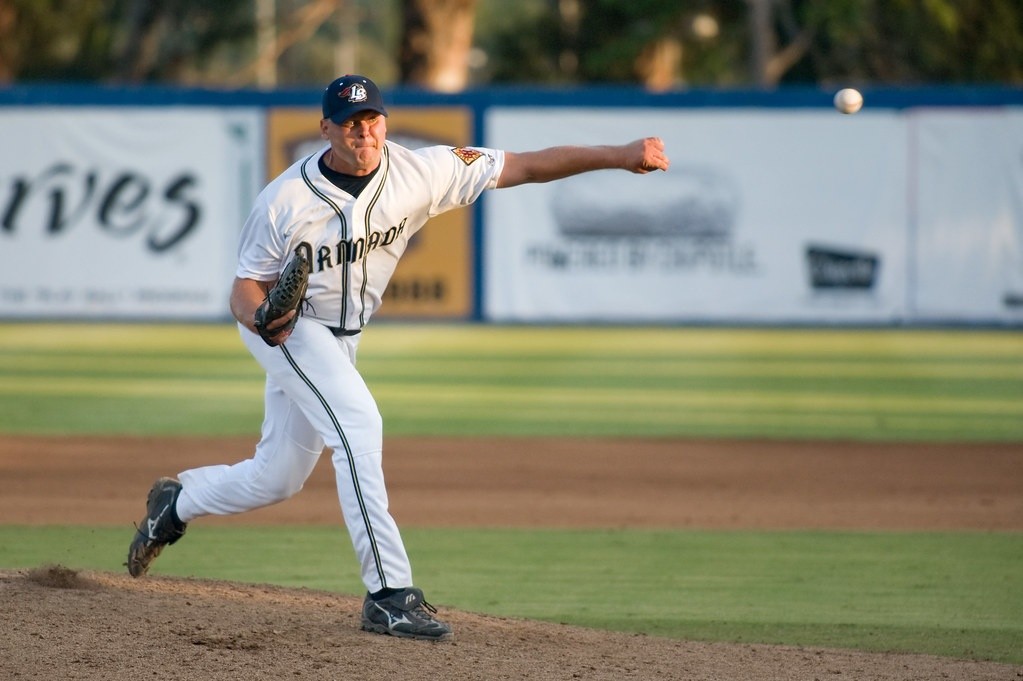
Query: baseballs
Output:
[[833, 88, 863, 114]]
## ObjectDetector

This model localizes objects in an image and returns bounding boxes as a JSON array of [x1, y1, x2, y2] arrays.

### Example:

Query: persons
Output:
[[128, 76, 669, 638], [636, 36, 682, 87]]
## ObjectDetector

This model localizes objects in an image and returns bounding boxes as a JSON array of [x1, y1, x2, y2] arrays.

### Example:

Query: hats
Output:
[[322, 74, 387, 125]]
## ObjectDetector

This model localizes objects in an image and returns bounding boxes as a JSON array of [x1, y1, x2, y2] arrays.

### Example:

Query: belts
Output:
[[328, 324, 361, 337]]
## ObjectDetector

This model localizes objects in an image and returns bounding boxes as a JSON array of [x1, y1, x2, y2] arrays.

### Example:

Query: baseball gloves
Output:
[[254, 249, 310, 347]]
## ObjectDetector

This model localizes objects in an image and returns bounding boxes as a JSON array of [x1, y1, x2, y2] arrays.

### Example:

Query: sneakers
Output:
[[359, 585, 453, 640], [127, 476, 186, 578]]
